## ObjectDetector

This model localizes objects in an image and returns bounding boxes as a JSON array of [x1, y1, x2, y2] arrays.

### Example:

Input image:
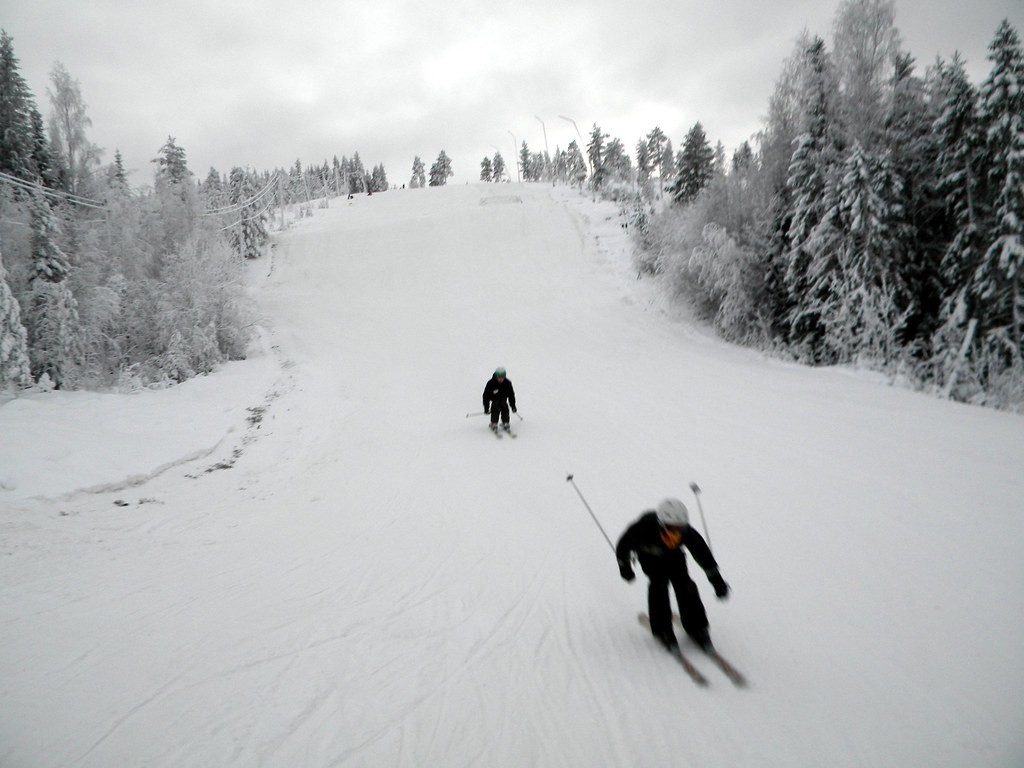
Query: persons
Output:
[[483, 367, 517, 433], [616, 499, 728, 655]]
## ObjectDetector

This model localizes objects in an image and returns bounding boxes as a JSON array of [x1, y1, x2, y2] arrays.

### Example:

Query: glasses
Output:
[[664, 522, 685, 534]]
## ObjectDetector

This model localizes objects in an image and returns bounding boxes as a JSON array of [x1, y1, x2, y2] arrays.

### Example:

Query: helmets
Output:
[[656, 499, 689, 525], [495, 367, 506, 375]]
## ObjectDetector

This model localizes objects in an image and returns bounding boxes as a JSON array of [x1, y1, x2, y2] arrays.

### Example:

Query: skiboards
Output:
[[500, 423, 519, 438], [697, 640, 750, 691], [488, 422, 505, 439], [637, 611, 712, 688]]
